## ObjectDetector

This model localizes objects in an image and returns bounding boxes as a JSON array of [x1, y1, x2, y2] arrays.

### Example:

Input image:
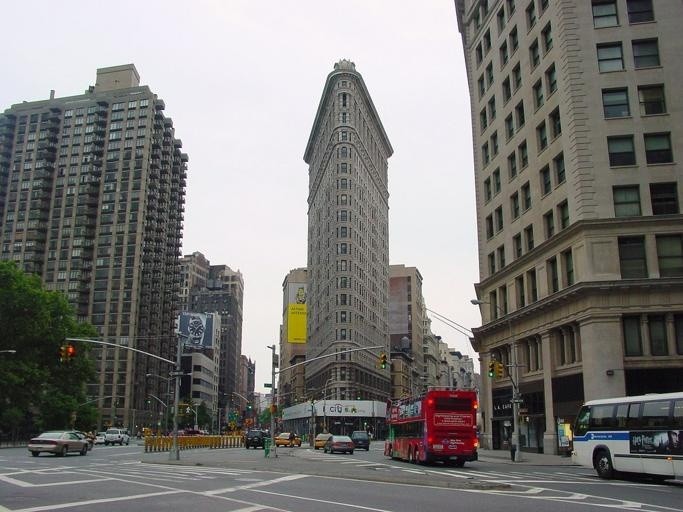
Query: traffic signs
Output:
[[263, 383, 272, 388]]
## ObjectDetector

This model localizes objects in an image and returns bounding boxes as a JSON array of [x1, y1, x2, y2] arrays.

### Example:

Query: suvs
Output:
[[244, 429, 269, 449]]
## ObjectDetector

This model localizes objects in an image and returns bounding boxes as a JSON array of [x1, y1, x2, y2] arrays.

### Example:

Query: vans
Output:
[[351, 430, 370, 451], [103, 428, 130, 447]]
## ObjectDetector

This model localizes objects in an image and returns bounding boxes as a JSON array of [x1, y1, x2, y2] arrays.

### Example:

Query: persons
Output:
[[289, 430, 295, 448], [667, 431, 681, 449]]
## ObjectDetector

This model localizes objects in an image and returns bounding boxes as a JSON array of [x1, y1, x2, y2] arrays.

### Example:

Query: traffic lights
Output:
[[65, 345, 73, 362], [494, 361, 503, 380], [59, 345, 65, 363], [185, 406, 191, 414], [269, 403, 277, 414], [379, 352, 387, 370], [146, 396, 151, 405], [247, 404, 253, 410], [486, 360, 495, 378], [356, 389, 361, 400]]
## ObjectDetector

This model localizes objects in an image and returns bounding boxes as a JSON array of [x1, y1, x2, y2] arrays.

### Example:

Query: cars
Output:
[[75, 430, 93, 451], [322, 434, 355, 455], [162, 428, 213, 437], [23, 430, 88, 457], [313, 433, 333, 450], [93, 432, 107, 446], [274, 432, 302, 448]]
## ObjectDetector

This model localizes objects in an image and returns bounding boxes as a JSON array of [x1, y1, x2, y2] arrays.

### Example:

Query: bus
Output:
[[568, 390, 682, 480], [382, 389, 480, 468]]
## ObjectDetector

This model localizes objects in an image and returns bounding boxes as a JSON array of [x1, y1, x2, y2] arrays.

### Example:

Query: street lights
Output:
[[0, 349, 17, 355], [320, 378, 334, 433], [145, 372, 193, 435], [470, 299, 523, 464]]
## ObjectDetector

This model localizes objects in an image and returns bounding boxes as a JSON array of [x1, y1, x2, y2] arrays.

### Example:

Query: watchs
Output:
[[187, 316, 204, 344]]
